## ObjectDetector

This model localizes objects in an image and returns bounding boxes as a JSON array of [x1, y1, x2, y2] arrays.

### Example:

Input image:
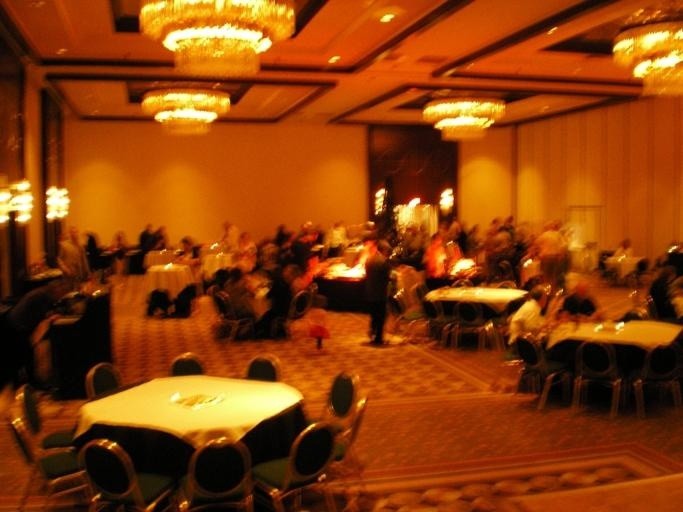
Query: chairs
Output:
[[248, 354, 280, 382], [320, 369, 360, 419], [77, 438, 174, 511], [254, 422, 335, 512], [174, 435, 254, 511], [85, 362, 118, 400], [420, 298, 454, 349], [170, 352, 206, 376], [388, 291, 424, 341], [633, 344, 678, 419], [571, 338, 625, 419], [626, 257, 651, 287], [330, 397, 369, 475], [515, 332, 562, 410], [450, 299, 487, 351], [11, 418, 87, 512], [15, 384, 75, 446], [280, 288, 311, 345], [216, 291, 254, 344]]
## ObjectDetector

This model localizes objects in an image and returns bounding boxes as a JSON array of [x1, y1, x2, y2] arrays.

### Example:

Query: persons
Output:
[[328, 215, 470, 350], [214, 216, 323, 339], [580, 236, 683, 285], [111, 222, 207, 299], [473, 215, 575, 314], [0, 276, 74, 429], [84, 232, 107, 284], [57, 225, 90, 281], [504, 271, 683, 395]]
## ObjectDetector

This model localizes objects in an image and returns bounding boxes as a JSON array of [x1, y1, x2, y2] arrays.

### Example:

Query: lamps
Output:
[[140, 0, 297, 77], [423, 98, 506, 140], [140, 89, 230, 137], [614, 21, 683, 97]]
